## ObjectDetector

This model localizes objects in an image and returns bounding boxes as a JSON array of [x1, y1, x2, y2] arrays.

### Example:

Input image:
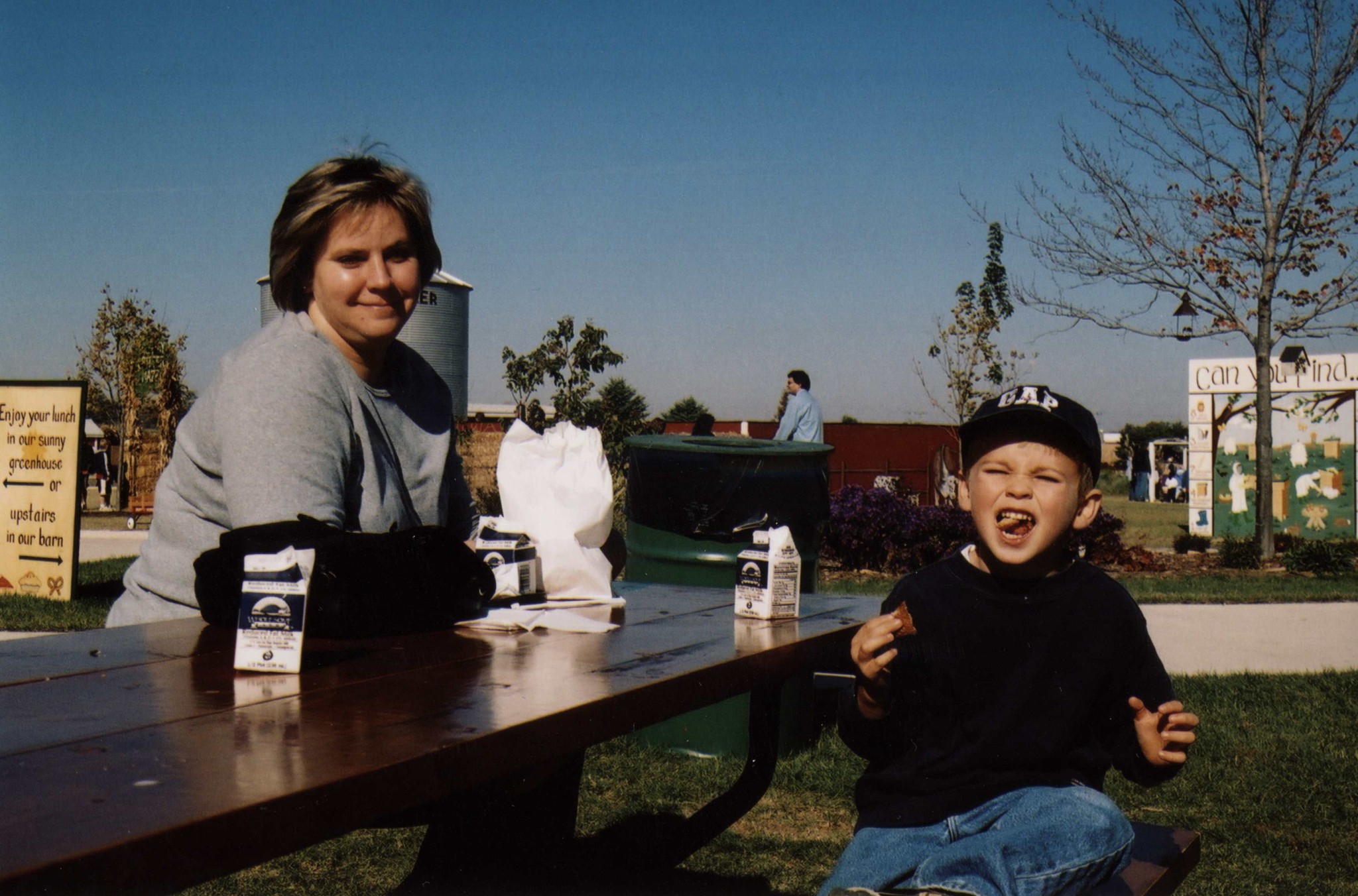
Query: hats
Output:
[[958, 384, 1101, 489]]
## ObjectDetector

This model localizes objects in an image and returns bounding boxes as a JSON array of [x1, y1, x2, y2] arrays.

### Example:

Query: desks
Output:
[[0, 582, 884, 896]]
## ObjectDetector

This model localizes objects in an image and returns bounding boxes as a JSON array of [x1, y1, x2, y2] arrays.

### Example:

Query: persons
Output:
[[1128, 444, 1189, 502], [105, 138, 582, 896], [690, 413, 715, 437], [774, 369, 823, 447], [81, 438, 113, 511], [819, 386, 1203, 896]]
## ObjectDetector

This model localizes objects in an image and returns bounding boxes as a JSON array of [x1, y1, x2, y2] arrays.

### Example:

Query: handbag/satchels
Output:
[[193, 514, 497, 637]]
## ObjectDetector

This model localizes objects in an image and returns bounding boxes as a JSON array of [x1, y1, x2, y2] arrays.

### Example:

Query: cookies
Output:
[[891, 601, 917, 636]]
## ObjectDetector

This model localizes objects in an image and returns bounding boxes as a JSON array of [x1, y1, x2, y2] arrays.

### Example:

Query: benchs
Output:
[[1087, 822, 1201, 896]]
